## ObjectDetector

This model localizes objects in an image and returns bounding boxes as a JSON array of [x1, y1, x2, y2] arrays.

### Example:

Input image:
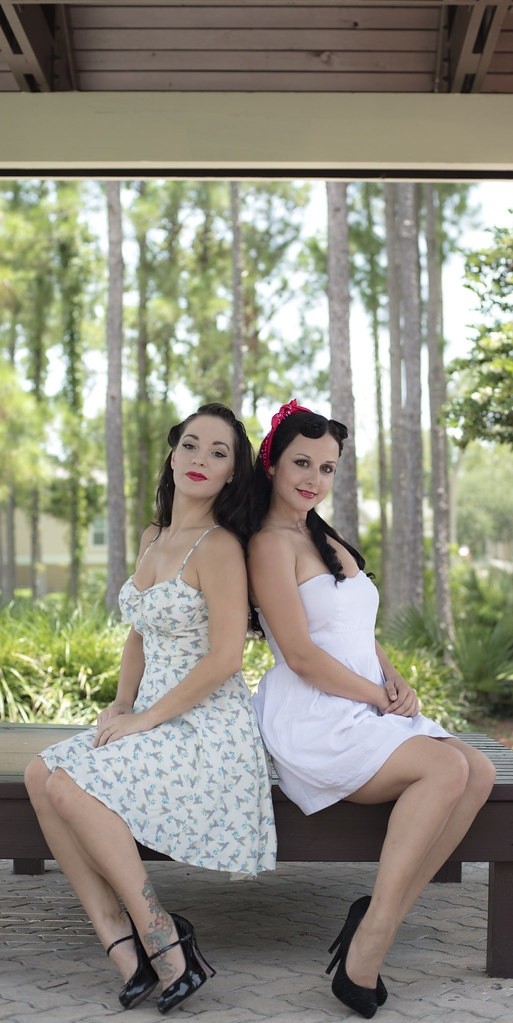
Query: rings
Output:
[[105, 728, 111, 735]]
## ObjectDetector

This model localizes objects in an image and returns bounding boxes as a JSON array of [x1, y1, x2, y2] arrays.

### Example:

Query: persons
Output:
[[244, 411, 496, 1019], [22, 404, 256, 1014]]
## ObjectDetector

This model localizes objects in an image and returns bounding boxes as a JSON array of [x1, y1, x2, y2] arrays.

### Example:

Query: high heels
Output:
[[325, 923, 379, 1019], [107, 911, 160, 1009], [329, 895, 388, 1005], [145, 912, 216, 1015]]
